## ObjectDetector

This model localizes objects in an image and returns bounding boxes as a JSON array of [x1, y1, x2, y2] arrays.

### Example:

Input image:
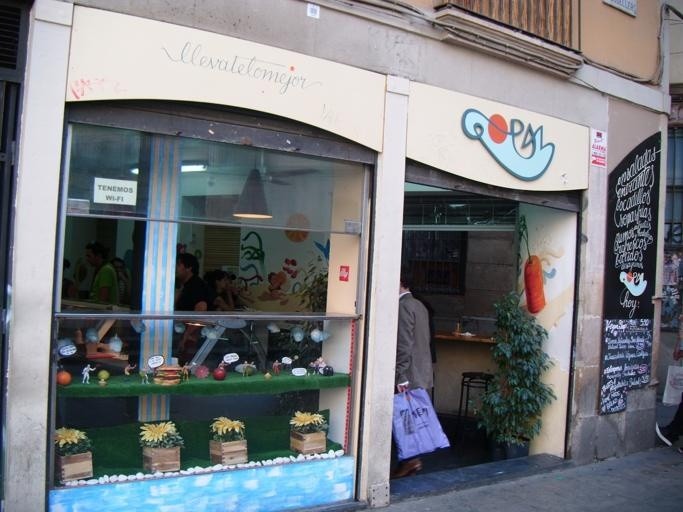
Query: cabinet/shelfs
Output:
[[48, 310, 361, 490]]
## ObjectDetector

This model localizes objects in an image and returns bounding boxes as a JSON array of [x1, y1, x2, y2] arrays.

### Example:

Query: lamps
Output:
[[130, 160, 208, 176], [234, 148, 272, 217]]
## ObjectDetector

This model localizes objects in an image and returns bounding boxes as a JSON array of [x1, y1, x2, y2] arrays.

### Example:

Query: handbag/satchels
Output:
[[662, 365, 683, 407]]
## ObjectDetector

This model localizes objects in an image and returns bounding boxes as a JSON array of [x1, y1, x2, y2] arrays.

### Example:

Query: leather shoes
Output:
[[391, 458, 422, 478], [390, 463, 417, 476]]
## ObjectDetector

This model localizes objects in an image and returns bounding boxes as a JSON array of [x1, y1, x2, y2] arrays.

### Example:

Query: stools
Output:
[[458, 372, 496, 437]]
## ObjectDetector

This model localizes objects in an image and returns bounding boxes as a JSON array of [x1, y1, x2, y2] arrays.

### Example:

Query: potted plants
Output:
[[489, 289, 556, 461]]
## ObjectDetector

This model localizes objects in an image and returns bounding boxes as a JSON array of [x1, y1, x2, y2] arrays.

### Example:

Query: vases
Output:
[[56, 451, 93, 482], [209, 438, 248, 464], [142, 447, 180, 473], [289, 430, 327, 454]]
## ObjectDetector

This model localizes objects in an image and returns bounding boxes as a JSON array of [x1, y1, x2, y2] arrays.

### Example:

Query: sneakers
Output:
[[656, 422, 678, 447]]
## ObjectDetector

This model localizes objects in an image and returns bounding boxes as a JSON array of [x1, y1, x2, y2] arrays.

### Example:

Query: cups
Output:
[[172, 358, 180, 367]]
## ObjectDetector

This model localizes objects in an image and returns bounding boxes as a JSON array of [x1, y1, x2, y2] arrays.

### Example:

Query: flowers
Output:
[[209, 417, 246, 440], [289, 410, 330, 432], [54, 427, 92, 455], [137, 422, 185, 449]]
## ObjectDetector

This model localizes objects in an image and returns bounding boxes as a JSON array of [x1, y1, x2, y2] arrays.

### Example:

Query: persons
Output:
[[392, 265, 434, 479], [655, 262, 683, 453], [58, 242, 134, 362], [268, 271, 294, 296], [175, 241, 250, 354]]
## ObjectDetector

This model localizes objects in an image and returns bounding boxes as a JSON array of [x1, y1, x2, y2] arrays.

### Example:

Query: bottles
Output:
[[405, 231, 457, 259], [432, 200, 445, 223], [455, 313, 460, 332], [406, 260, 465, 295]]
